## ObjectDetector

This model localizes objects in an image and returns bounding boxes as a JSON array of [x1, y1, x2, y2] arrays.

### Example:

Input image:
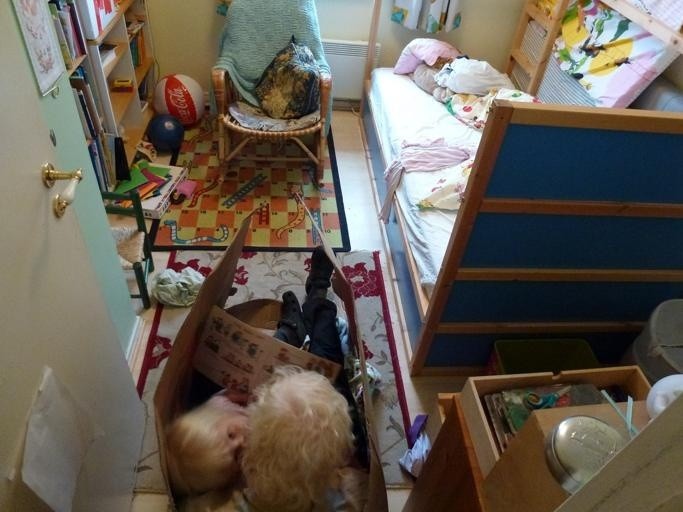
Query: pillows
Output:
[[253, 34, 320, 120], [392, 38, 462, 75]]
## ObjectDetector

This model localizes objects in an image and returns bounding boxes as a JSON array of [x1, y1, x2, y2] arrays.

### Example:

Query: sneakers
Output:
[[278, 291, 306, 347], [306, 246, 336, 295]]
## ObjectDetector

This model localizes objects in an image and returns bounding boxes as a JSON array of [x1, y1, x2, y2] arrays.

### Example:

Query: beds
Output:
[[357, 58, 682, 378]]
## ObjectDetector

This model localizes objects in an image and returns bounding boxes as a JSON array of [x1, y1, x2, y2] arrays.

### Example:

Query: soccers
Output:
[[147, 115, 184, 153]]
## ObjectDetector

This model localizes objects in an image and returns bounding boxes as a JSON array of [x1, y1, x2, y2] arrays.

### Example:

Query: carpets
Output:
[[131, 248, 417, 495], [141, 104, 352, 253]]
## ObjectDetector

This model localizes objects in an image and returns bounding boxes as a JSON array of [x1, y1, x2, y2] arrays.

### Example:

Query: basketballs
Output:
[[153, 73, 205, 128]]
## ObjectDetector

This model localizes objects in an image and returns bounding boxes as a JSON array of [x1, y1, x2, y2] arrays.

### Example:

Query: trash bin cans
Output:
[[479, 339, 600, 376]]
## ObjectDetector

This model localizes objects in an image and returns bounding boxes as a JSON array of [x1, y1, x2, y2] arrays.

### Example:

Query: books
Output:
[[192, 304, 343, 395], [47, 0, 148, 194]]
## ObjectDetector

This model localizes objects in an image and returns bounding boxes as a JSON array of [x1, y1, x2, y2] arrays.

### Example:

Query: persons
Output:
[[180, 248, 370, 512], [165, 381, 253, 497]]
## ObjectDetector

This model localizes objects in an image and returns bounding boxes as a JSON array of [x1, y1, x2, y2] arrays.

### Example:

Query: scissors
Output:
[[523, 385, 572, 410]]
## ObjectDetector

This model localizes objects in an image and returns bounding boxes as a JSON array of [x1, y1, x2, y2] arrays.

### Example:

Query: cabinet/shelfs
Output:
[[44, 0, 119, 209], [75, 1, 157, 168], [399, 364, 682, 512]]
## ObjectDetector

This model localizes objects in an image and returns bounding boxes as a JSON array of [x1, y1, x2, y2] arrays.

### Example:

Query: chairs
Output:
[[209, 0, 335, 173], [100, 189, 155, 311]]
